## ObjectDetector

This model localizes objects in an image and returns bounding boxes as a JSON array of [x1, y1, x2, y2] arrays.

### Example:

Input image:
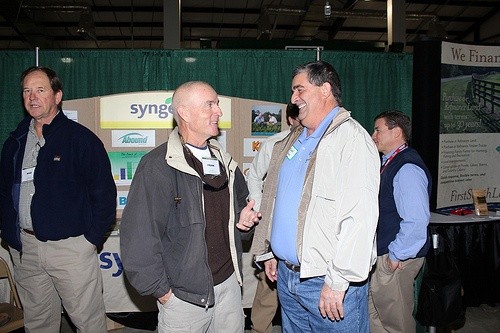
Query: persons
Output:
[[246, 102, 308, 333], [369, 110, 432, 333], [250, 61, 381, 332], [120, 80, 261, 333], [0, 66, 118, 333]]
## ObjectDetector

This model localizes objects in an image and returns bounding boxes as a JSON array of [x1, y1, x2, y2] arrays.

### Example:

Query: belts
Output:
[[282, 260, 300, 273], [19, 228, 34, 236]]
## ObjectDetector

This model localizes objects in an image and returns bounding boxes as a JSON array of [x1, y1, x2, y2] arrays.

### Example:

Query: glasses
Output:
[[191, 157, 229, 191]]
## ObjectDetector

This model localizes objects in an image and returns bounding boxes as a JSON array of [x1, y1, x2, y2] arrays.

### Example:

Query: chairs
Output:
[[0, 256, 25, 333]]
